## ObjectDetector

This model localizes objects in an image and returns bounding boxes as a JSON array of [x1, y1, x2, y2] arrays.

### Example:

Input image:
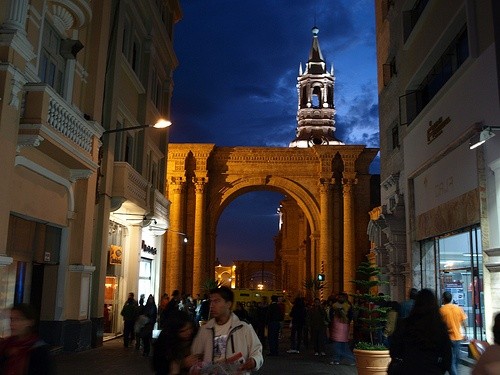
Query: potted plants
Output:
[[339, 255, 393, 375]]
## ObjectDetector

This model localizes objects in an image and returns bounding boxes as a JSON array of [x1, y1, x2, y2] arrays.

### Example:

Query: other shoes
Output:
[[320, 352, 327, 356], [287, 349, 296, 352], [297, 351, 300, 354], [314, 352, 319, 356]]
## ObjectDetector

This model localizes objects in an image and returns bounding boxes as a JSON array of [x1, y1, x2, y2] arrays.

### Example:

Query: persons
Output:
[[0, 304, 58, 375], [120, 288, 419, 364], [150, 311, 201, 375], [387, 288, 452, 375], [439, 292, 464, 375], [189, 286, 264, 375], [471, 313, 500, 375]]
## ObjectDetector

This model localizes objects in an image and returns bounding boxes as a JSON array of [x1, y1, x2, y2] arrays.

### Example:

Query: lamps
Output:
[[113, 213, 151, 227]]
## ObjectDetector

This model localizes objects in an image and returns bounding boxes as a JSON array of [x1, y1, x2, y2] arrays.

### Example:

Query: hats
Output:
[[172, 290, 179, 296]]
